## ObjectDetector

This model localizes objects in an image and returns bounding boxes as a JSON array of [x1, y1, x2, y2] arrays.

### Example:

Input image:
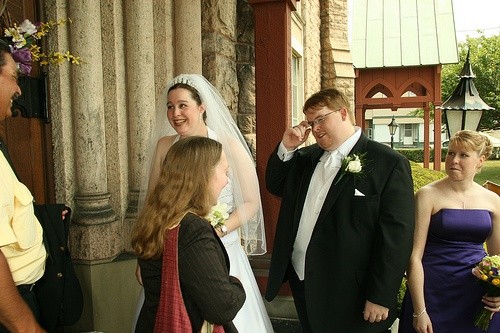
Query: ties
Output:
[[323, 150, 338, 184]]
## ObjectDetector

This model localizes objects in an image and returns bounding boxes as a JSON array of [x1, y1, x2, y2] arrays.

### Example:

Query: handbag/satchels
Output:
[[150, 225, 225, 333]]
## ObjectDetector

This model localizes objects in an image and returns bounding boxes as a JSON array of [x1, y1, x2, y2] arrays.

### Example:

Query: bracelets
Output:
[[413, 307, 426, 318], [214, 223, 227, 237]]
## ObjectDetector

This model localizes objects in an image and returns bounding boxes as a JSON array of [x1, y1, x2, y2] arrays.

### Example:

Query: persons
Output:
[[128, 136, 246, 333], [265, 88, 415, 333], [398, 130, 500, 333], [133, 74, 274, 333], [0, 38, 68, 333]]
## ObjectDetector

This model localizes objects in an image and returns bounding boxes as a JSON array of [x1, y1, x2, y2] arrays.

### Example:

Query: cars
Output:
[[430, 132, 500, 147]]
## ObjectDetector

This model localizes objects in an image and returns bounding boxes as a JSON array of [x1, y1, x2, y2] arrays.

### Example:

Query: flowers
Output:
[[3, 18, 88, 75], [472, 255, 500, 333], [335, 152, 374, 185], [203, 200, 231, 227]]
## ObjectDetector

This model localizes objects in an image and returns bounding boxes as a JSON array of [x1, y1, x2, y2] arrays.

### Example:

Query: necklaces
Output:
[[446, 178, 473, 209]]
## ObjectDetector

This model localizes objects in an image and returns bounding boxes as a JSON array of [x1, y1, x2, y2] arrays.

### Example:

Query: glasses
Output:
[[308, 108, 341, 128]]
